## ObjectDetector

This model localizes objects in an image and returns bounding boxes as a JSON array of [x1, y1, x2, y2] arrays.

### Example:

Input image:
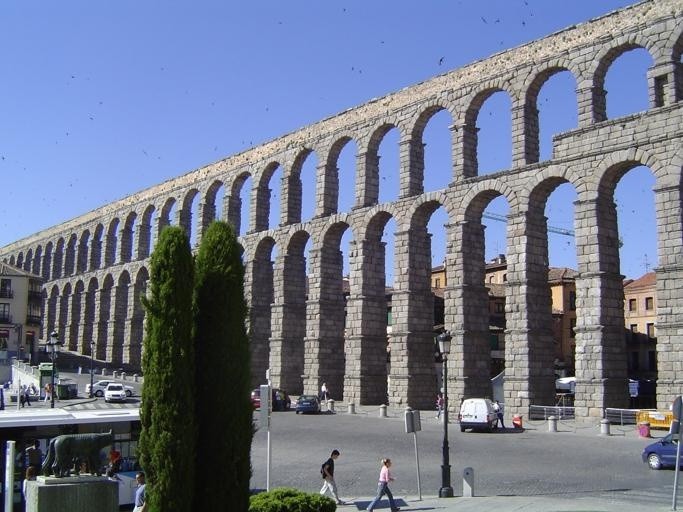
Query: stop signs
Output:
[[512, 418, 522, 427]]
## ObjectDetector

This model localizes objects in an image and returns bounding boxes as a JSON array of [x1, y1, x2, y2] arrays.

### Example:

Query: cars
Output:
[[103, 383, 126, 402], [554, 376, 575, 390], [294, 395, 320, 414], [84, 379, 135, 397], [641, 433, 683, 469], [251, 388, 291, 410]]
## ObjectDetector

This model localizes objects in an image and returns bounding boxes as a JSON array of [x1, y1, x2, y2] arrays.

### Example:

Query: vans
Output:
[[457, 398, 498, 432]]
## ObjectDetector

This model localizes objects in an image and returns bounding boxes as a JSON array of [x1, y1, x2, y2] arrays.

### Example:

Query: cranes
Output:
[[481, 210, 624, 249]]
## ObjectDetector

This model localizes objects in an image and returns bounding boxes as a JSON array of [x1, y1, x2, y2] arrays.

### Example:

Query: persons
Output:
[[434, 393, 444, 419], [25, 387, 31, 406], [319, 450, 345, 504], [44, 382, 52, 404], [110, 446, 122, 472], [493, 401, 505, 429], [14, 438, 42, 476], [318, 382, 328, 401], [21, 466, 37, 499], [133, 472, 148, 512], [20, 386, 25, 407], [366, 457, 400, 512]]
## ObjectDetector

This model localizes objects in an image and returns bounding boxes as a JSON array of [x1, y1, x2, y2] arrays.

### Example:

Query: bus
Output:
[[0, 408, 143, 509]]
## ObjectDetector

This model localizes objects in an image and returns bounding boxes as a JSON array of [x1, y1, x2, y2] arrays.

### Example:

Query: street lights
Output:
[[11, 323, 24, 359], [88, 340, 95, 398], [437, 329, 454, 498], [45, 330, 61, 408]]
